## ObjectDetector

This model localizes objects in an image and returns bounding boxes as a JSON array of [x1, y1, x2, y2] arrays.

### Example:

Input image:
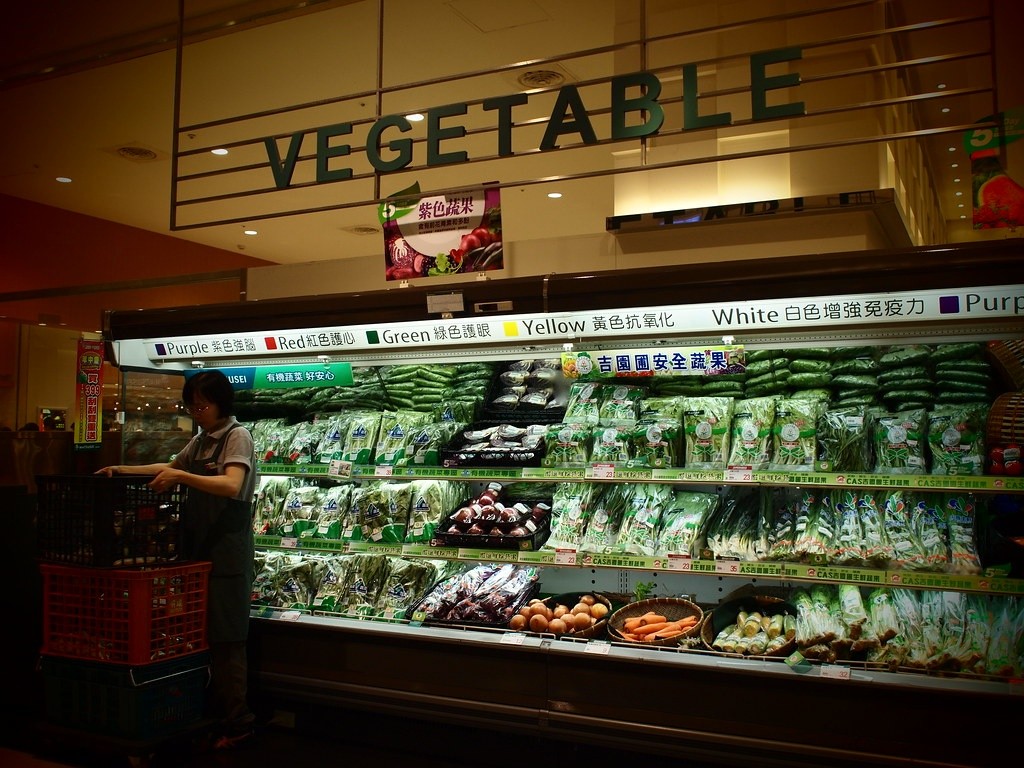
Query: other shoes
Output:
[[214, 727, 256, 752]]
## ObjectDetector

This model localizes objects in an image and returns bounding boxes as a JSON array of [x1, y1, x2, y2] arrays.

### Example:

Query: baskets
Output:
[[433, 498, 552, 551], [607, 598, 704, 647], [438, 361, 567, 467], [700, 596, 797, 661], [541, 592, 612, 639]]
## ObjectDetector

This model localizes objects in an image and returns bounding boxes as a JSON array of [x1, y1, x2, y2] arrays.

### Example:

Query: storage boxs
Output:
[[986, 392, 1024, 448], [482, 360, 565, 419], [439, 417, 561, 467], [984, 340, 1024, 391], [34, 559, 214, 667], [34, 473, 188, 570], [436, 497, 552, 552], [405, 576, 542, 634], [39, 650, 208, 742]]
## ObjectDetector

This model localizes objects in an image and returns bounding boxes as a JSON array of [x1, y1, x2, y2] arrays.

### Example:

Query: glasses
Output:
[[185, 402, 214, 415]]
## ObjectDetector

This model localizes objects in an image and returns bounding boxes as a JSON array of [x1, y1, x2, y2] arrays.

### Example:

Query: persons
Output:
[[1, 417, 74, 431], [93, 369, 258, 751]]
